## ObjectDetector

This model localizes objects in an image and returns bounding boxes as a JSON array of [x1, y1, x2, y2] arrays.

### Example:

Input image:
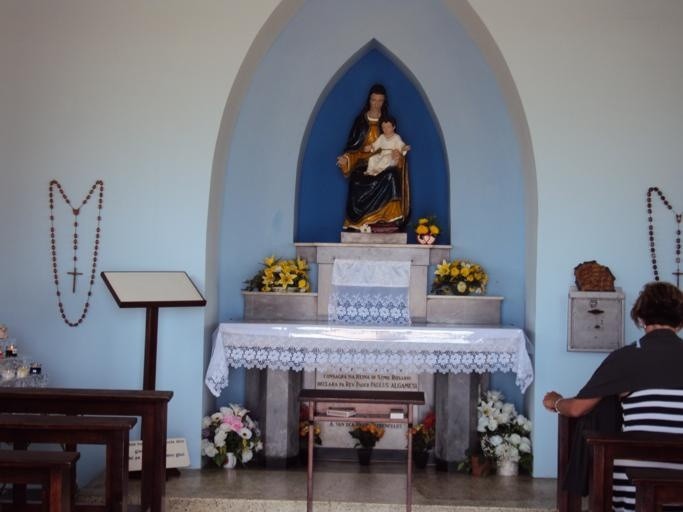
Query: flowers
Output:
[[347, 424, 379, 445], [407, 410, 438, 447], [478, 389, 533, 461], [298, 403, 322, 443], [239, 251, 313, 295], [411, 212, 443, 244], [199, 404, 263, 465], [427, 257, 488, 294]]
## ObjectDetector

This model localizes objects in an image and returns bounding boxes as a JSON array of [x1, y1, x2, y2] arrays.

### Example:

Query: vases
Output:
[[219, 452, 237, 469], [470, 452, 493, 478], [493, 455, 520, 476], [354, 448, 372, 466], [412, 447, 427, 468]]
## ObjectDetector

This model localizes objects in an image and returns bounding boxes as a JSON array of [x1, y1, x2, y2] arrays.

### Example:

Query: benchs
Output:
[[556, 397, 683, 512], [0, 386, 174, 511]]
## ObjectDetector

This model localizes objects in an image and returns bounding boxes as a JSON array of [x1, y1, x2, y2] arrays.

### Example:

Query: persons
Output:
[[541, 280, 683, 511], [336, 81, 412, 234], [364, 116, 411, 177]]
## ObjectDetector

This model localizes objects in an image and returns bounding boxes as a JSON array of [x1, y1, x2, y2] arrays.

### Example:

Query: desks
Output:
[[297, 386, 426, 512], [204, 320, 537, 472]]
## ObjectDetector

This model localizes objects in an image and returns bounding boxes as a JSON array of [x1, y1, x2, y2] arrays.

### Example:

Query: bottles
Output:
[[1, 367, 15, 380], [5, 344, 18, 359], [30, 362, 42, 375], [0, 324, 9, 340], [16, 367, 29, 378]]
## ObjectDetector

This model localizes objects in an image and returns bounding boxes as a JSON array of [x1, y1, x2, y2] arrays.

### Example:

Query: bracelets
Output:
[[554, 397, 562, 413]]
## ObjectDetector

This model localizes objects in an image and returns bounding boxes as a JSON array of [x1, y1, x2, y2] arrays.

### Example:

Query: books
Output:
[[326, 408, 357, 419], [388, 408, 404, 419]]
